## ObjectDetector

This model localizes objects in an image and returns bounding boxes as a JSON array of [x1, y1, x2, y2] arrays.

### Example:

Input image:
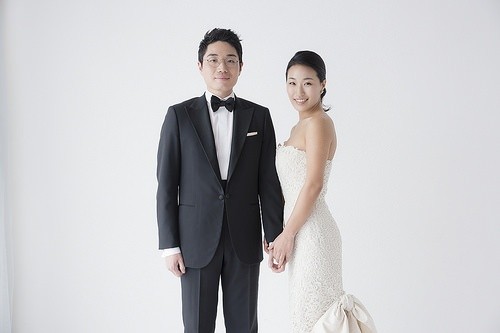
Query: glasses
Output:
[[206, 56, 238, 68]]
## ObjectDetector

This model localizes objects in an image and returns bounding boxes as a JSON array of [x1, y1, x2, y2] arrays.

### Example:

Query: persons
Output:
[[156, 27, 285, 333], [264, 51, 378, 333]]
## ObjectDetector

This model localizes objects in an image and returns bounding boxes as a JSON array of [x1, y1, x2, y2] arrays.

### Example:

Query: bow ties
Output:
[[211, 95, 235, 112]]
